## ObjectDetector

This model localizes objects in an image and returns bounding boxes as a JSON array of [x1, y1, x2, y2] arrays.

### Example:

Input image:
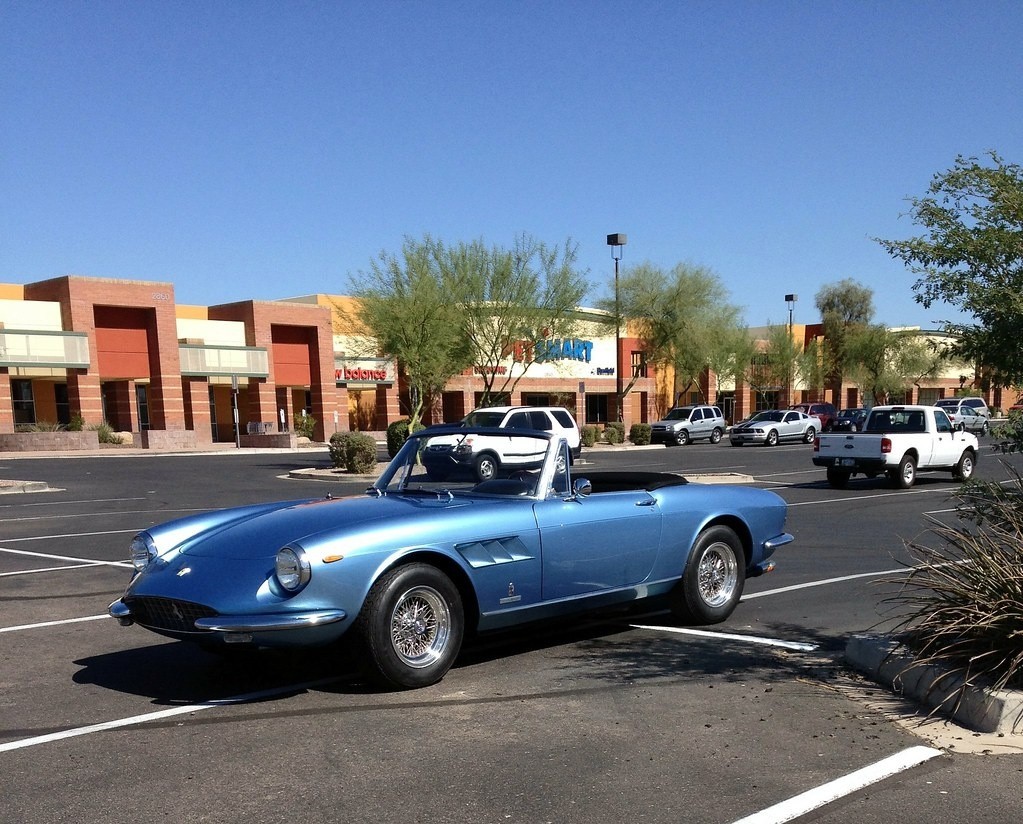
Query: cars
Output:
[[736, 410, 763, 425], [1009, 397, 1023, 413], [729, 410, 823, 446], [941, 406, 991, 434], [828, 408, 871, 433]]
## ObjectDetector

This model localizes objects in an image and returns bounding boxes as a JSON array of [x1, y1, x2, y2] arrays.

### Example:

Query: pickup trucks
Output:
[[811, 405, 980, 491]]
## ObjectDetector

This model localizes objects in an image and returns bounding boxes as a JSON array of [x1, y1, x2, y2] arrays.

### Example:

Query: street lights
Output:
[[785, 294, 799, 405], [606, 233, 627, 420]]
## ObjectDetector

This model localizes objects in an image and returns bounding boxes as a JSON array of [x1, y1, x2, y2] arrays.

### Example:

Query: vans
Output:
[[788, 401, 837, 433], [935, 395, 991, 416]]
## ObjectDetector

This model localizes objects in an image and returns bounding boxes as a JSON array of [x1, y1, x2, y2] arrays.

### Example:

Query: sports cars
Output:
[[105, 422, 794, 691]]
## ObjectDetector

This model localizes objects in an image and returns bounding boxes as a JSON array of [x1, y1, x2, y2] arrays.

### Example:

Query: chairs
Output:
[[903, 413, 923, 430], [471, 480, 534, 494], [871, 415, 894, 430]]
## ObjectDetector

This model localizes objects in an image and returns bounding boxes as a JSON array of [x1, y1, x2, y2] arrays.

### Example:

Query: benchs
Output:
[[506, 470, 690, 492]]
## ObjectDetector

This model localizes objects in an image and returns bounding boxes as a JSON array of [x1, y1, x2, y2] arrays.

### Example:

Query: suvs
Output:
[[421, 406, 583, 481], [650, 404, 727, 446]]
